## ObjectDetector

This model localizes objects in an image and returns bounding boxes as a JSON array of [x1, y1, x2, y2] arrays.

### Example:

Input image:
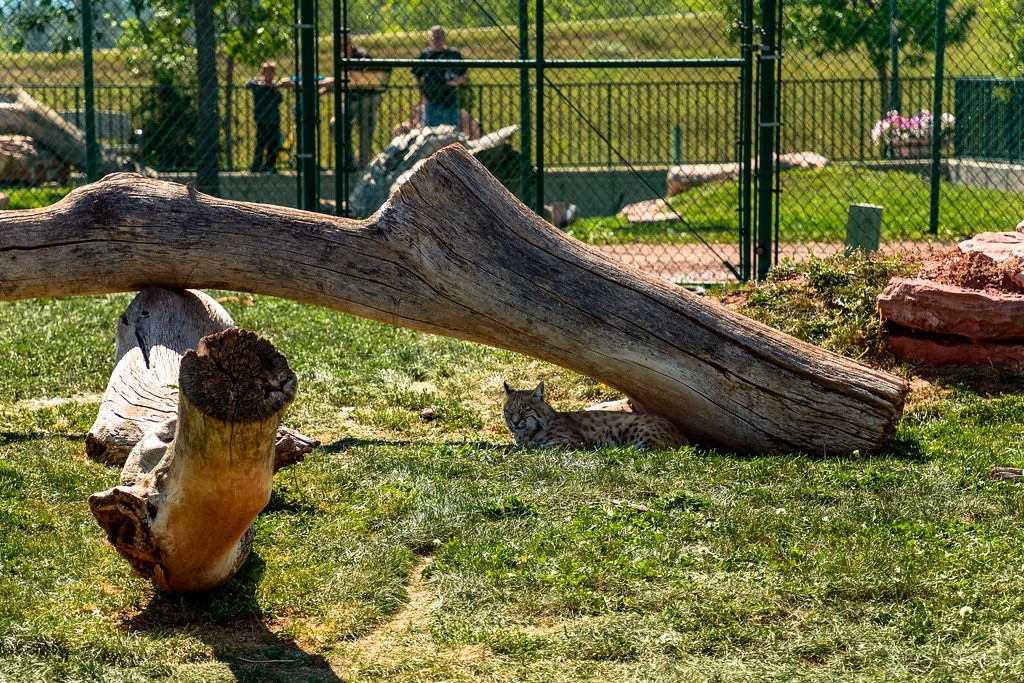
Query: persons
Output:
[[411, 25, 470, 132], [243, 62, 283, 173], [278, 75, 334, 167], [329, 28, 392, 173]]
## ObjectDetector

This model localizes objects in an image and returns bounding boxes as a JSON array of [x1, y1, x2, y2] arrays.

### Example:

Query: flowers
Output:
[[872, 109, 957, 147]]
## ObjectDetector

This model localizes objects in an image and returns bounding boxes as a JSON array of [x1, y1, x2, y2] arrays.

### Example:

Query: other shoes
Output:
[[265, 165, 276, 173], [249, 165, 264, 172]]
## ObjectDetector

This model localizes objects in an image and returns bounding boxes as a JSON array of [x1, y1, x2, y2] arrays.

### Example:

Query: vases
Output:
[[885, 141, 934, 159]]
